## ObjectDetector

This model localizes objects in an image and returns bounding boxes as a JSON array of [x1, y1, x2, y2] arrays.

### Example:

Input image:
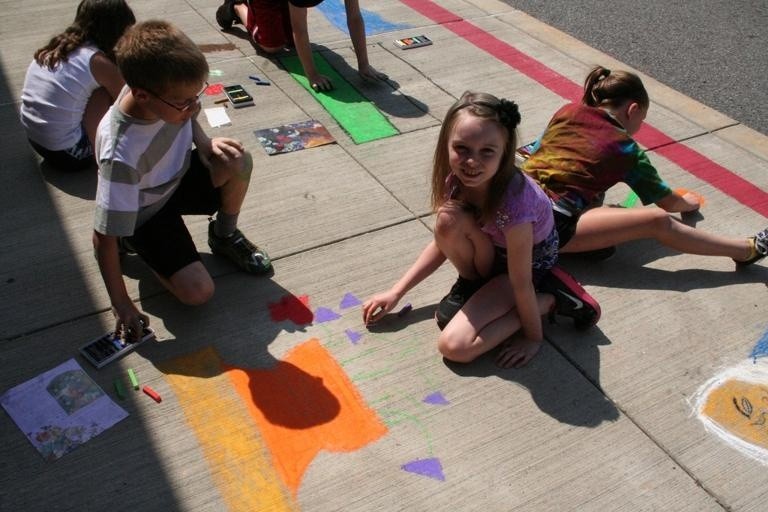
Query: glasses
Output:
[[146, 81, 210, 113]]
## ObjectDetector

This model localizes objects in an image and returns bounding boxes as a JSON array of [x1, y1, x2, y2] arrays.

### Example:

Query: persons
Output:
[[361, 90, 601, 369], [19, 1, 136, 174], [93, 20, 271, 348], [216, 0, 388, 93], [521, 64, 768, 266]]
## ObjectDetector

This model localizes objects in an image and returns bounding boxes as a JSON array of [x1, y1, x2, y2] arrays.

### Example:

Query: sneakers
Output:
[[215, 0, 245, 30], [732, 226, 768, 268], [434, 277, 482, 326], [206, 216, 272, 275], [534, 265, 602, 330]]
